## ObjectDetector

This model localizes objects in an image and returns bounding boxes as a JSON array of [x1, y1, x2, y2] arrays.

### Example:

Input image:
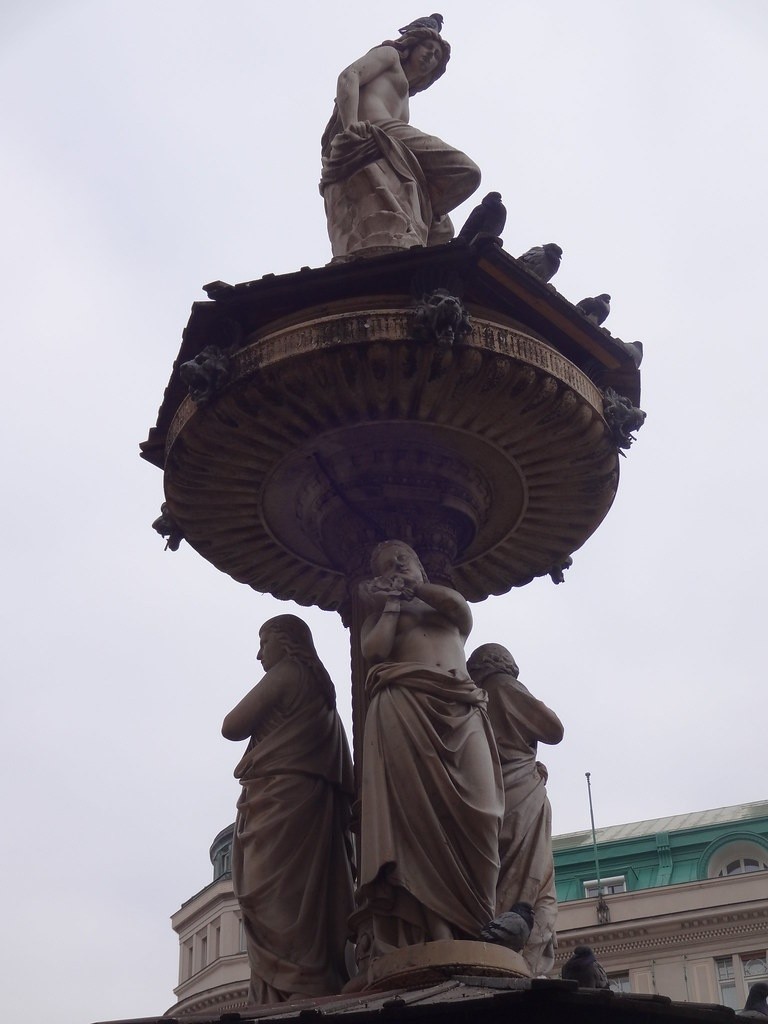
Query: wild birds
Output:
[[479, 902, 611, 989], [744, 981, 768, 1016], [453, 189, 645, 367]]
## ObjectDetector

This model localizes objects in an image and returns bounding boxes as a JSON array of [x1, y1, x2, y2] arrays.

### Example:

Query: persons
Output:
[[471, 642, 563, 973], [220, 614, 358, 1003], [354, 539, 505, 938], [321, 12, 479, 244]]
